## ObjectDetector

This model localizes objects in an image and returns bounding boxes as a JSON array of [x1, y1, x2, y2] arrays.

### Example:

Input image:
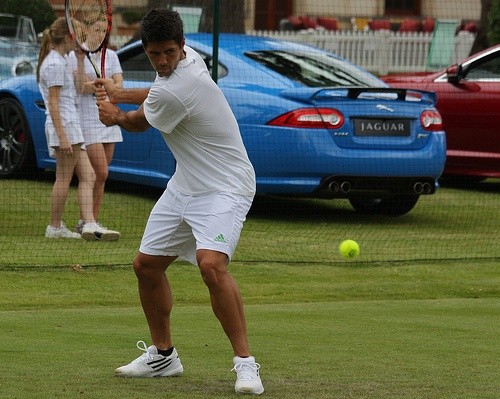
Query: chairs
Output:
[[288, 15, 478, 34]]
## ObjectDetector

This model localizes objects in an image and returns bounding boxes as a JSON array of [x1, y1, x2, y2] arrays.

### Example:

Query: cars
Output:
[[378, 44, 500, 190]]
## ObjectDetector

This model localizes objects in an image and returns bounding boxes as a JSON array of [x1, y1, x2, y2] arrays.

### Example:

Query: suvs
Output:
[[0, 14, 43, 81]]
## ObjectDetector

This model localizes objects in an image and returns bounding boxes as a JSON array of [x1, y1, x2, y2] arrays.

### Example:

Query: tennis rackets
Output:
[[65, 0, 118, 127]]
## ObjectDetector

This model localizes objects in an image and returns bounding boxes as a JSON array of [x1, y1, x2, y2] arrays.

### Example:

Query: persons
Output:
[[93, 7, 264, 394], [69, 11, 123, 232], [36, 16, 120, 241]]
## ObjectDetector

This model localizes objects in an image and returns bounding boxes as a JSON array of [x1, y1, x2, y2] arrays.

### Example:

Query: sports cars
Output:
[[0, 32, 448, 218]]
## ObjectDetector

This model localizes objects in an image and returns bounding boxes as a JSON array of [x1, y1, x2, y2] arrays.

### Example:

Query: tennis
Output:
[[340, 239, 359, 258]]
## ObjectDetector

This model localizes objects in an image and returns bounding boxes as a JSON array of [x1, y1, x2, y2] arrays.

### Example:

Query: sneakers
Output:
[[114, 339, 183, 377], [45, 220, 81, 239], [230, 356, 264, 395], [81, 223, 121, 241]]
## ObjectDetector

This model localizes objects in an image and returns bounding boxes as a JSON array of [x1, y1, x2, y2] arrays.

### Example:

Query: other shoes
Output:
[[75, 222, 107, 235]]
